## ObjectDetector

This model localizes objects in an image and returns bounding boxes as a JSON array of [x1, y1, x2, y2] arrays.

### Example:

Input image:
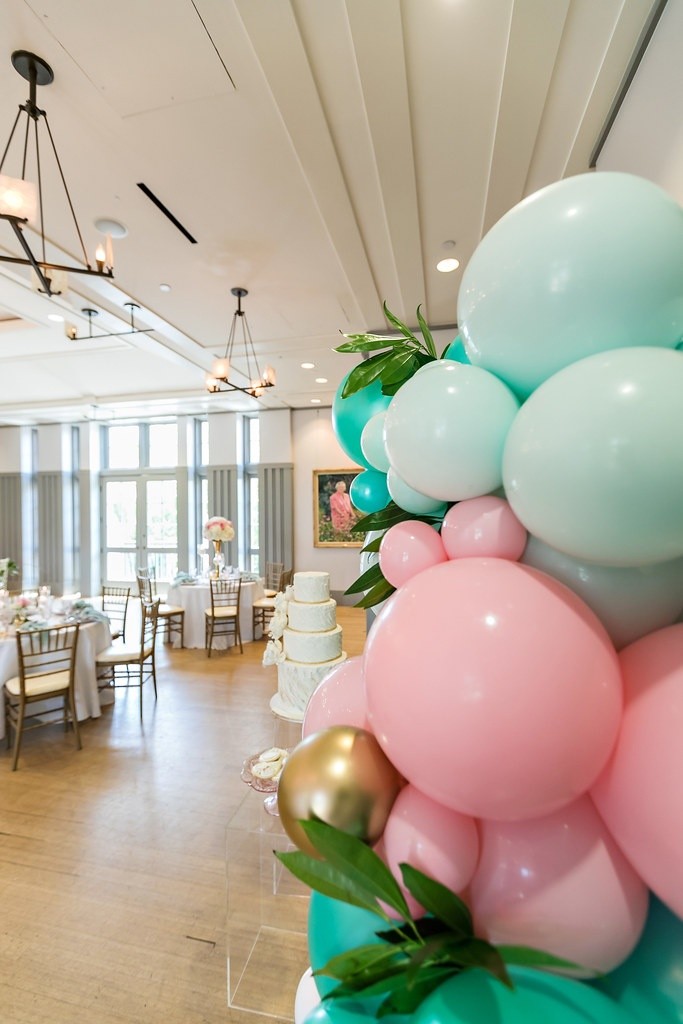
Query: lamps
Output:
[[0, 51, 114, 299], [64, 302, 154, 340], [206, 288, 276, 398]]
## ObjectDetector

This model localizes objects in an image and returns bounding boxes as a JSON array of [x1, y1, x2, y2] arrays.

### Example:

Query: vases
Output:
[[214, 540, 222, 578]]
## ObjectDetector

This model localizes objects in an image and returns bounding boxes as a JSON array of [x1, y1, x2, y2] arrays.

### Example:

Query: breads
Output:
[[251, 747, 290, 782]]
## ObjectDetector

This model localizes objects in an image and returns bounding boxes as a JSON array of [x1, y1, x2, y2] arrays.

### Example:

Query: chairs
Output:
[[251, 567, 292, 642], [95, 597, 160, 717], [264, 562, 284, 598], [3, 620, 82, 770], [138, 565, 165, 631], [101, 586, 130, 665], [138, 576, 184, 649], [203, 577, 243, 657]]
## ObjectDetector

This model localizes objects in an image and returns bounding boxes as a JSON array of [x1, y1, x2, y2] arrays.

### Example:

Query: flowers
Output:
[[203, 517, 234, 542]]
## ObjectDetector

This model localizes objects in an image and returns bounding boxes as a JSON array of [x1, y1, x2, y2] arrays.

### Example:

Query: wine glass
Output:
[[61, 599, 72, 623], [0, 608, 14, 640], [37, 596, 54, 625]]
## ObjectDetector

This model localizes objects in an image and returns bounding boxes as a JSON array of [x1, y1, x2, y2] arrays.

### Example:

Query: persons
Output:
[[330, 481, 356, 530]]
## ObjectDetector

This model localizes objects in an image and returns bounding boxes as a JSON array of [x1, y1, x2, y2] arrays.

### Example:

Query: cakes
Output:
[[262, 571, 347, 715]]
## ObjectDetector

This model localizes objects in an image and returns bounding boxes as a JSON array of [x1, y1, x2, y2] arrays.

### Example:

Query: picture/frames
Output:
[[313, 468, 369, 548]]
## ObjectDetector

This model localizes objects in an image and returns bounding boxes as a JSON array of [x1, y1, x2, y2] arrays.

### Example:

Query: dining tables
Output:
[[0, 600, 115, 740], [164, 572, 266, 651]]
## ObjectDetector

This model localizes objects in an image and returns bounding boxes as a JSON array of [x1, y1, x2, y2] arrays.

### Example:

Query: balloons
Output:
[[278, 168, 683, 1024]]
